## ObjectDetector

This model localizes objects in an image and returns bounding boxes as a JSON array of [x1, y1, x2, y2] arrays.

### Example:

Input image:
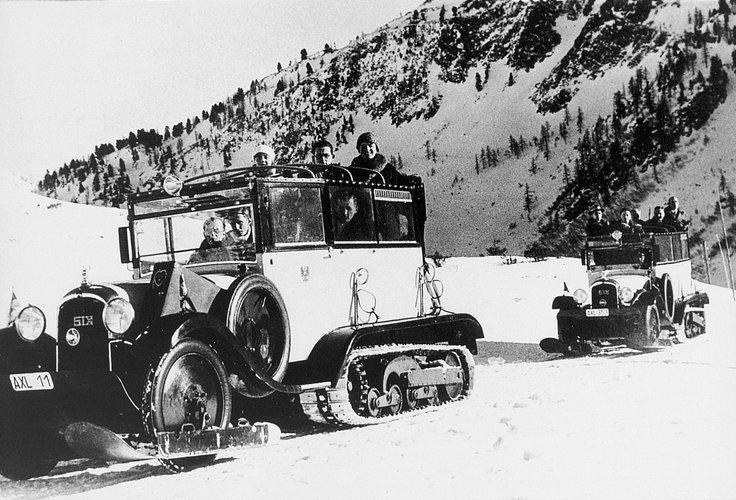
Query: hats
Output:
[[588, 205, 605, 216], [356, 132, 380, 153], [251, 146, 275, 162]]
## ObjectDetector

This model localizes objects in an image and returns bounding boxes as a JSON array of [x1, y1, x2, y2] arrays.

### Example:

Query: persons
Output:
[[299, 141, 344, 240], [186, 218, 243, 263], [226, 206, 254, 245], [332, 191, 371, 241], [251, 146, 298, 243], [583, 196, 692, 268], [347, 132, 403, 241]]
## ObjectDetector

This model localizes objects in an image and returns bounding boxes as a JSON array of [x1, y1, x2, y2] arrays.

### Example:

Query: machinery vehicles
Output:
[[539, 223, 709, 357], [0, 163, 485, 480]]
